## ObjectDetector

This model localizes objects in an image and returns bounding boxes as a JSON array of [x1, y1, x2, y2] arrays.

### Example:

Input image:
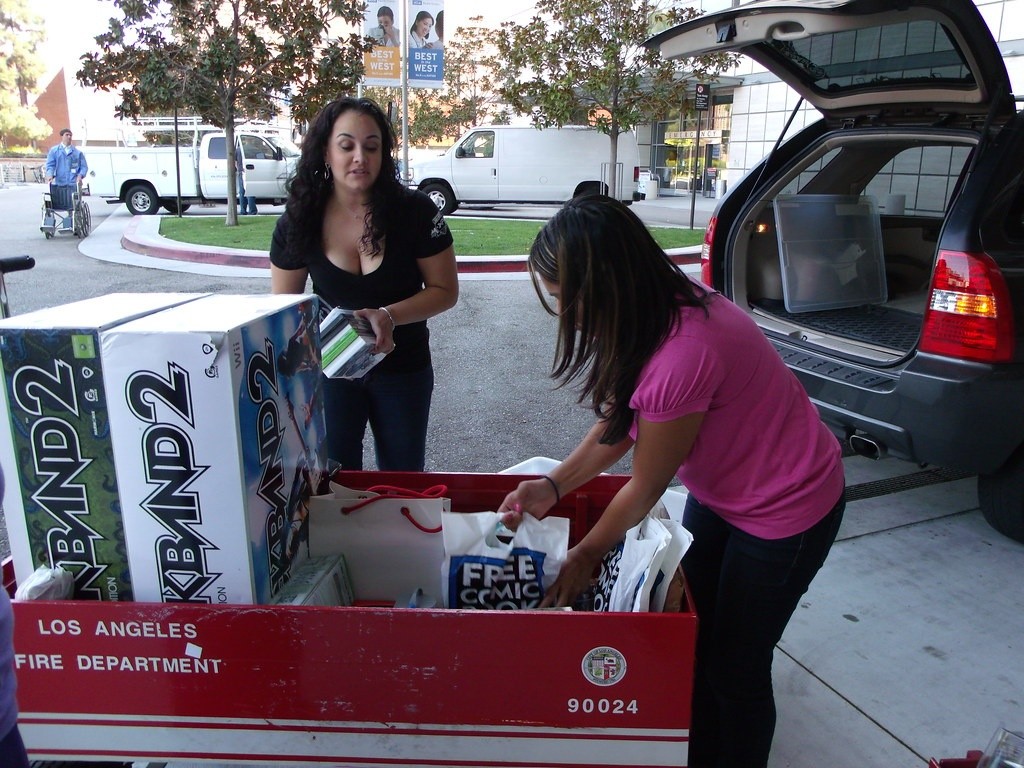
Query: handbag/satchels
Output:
[[305, 483, 694, 613]]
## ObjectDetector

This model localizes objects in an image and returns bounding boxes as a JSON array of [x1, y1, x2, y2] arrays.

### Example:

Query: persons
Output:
[[434, 11, 443, 49], [377, 6, 399, 47], [498, 195, 845, 768], [233, 136, 257, 215], [45, 129, 88, 235], [270, 99, 459, 472], [410, 11, 434, 48]]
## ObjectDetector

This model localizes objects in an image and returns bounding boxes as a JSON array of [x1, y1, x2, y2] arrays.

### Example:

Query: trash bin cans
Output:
[[644, 180, 657, 200], [715, 180, 726, 200]]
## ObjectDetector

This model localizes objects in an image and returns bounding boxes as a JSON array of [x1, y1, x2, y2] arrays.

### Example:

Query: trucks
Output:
[[396, 125, 645, 215], [74, 117, 301, 216]]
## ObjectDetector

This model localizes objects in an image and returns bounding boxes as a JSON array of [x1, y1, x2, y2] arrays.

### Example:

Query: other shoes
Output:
[[74, 228, 78, 236]]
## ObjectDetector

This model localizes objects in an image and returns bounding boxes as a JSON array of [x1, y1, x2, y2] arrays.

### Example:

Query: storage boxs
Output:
[[0, 293, 696, 767]]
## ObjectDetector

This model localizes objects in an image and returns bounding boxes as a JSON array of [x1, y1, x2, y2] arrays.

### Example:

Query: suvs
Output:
[[637, 1, 1023, 542]]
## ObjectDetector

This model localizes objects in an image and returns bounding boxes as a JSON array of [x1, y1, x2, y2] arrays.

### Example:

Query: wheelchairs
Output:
[[41, 177, 92, 239]]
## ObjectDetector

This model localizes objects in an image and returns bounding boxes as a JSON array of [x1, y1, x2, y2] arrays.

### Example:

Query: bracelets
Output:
[[378, 307, 395, 330], [542, 476, 559, 502]]
